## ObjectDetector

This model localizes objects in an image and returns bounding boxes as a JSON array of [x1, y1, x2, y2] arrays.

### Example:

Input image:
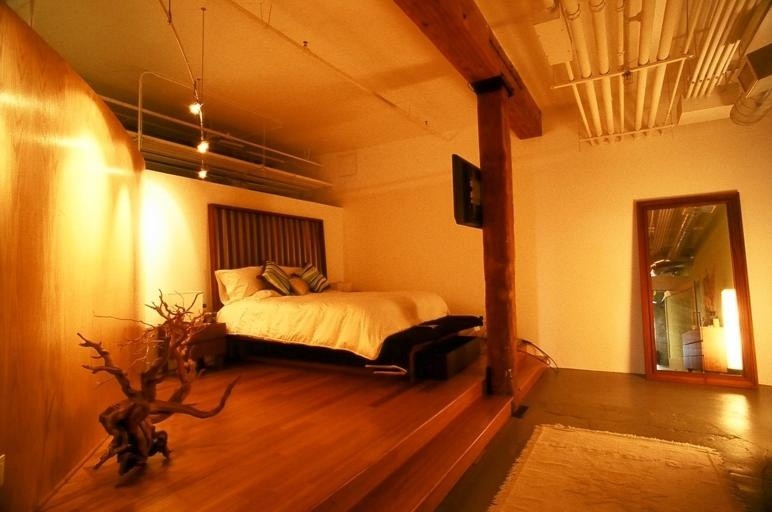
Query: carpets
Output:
[[479, 420, 748, 512]]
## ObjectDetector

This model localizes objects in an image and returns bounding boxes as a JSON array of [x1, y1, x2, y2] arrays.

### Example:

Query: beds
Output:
[[204, 202, 485, 384]]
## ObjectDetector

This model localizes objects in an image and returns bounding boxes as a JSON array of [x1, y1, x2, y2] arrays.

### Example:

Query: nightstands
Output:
[[155, 322, 225, 373]]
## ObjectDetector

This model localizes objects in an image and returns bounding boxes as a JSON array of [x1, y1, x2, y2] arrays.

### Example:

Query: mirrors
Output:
[[631, 188, 759, 392]]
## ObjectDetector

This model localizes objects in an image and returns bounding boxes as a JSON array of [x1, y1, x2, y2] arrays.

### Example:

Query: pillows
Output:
[[256, 255, 330, 297]]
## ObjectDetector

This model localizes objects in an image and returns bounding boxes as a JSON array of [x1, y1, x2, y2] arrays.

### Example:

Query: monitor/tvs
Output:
[[451, 153, 483, 230]]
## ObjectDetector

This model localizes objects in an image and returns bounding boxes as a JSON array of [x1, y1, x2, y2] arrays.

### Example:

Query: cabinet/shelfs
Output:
[[680, 326, 728, 373]]
